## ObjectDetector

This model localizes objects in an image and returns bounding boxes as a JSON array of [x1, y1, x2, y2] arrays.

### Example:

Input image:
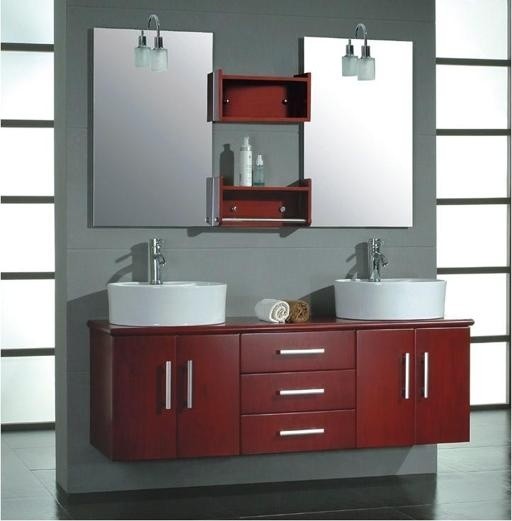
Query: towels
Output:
[[254, 297, 290, 323], [289, 299, 313, 326]]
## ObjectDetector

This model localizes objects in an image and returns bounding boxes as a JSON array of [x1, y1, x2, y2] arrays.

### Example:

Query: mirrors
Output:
[[89, 26, 226, 234], [299, 30, 416, 234]]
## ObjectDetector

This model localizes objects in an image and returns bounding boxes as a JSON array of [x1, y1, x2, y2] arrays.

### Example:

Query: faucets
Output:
[[366, 238, 389, 281], [148, 239, 165, 284]]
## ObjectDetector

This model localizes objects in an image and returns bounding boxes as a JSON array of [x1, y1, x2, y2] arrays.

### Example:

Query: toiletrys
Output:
[[254, 155, 263, 186], [254, 154, 265, 185]]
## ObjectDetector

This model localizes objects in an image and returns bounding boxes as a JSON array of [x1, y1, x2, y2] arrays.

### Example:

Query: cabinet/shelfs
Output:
[[79, 320, 477, 464], [207, 175, 315, 230], [209, 66, 314, 128]]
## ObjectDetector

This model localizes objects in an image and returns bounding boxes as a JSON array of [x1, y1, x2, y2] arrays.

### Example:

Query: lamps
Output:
[[134, 30, 151, 69], [348, 22, 379, 82], [340, 31, 360, 81], [145, 13, 169, 70]]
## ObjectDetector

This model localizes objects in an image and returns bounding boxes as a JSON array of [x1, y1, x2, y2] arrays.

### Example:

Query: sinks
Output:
[[107, 281, 227, 326], [334, 278, 447, 320]]
